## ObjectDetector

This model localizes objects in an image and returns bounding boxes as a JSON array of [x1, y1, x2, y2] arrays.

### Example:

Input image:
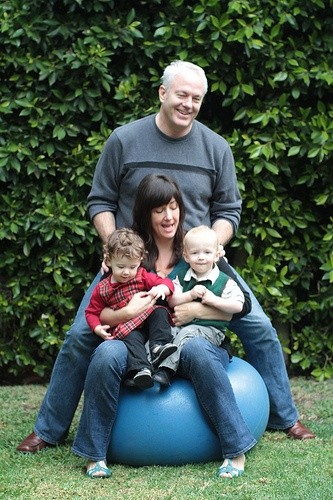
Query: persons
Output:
[[71, 174, 258, 480], [85, 229, 178, 391], [18, 60, 317, 452], [125, 225, 245, 389]]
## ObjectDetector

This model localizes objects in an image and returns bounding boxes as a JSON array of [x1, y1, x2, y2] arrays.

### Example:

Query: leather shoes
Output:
[[286, 419, 315, 440], [15, 432, 49, 453]]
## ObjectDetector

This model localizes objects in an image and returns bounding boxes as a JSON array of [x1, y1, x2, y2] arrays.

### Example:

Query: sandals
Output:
[[85, 459, 111, 478], [216, 452, 246, 479]]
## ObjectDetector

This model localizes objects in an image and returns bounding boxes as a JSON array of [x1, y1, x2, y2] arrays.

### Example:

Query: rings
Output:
[[172, 319, 175, 323]]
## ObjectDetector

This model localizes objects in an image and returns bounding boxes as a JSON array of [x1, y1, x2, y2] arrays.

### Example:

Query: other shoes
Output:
[[155, 369, 174, 386], [133, 369, 153, 386], [151, 343, 177, 367]]
